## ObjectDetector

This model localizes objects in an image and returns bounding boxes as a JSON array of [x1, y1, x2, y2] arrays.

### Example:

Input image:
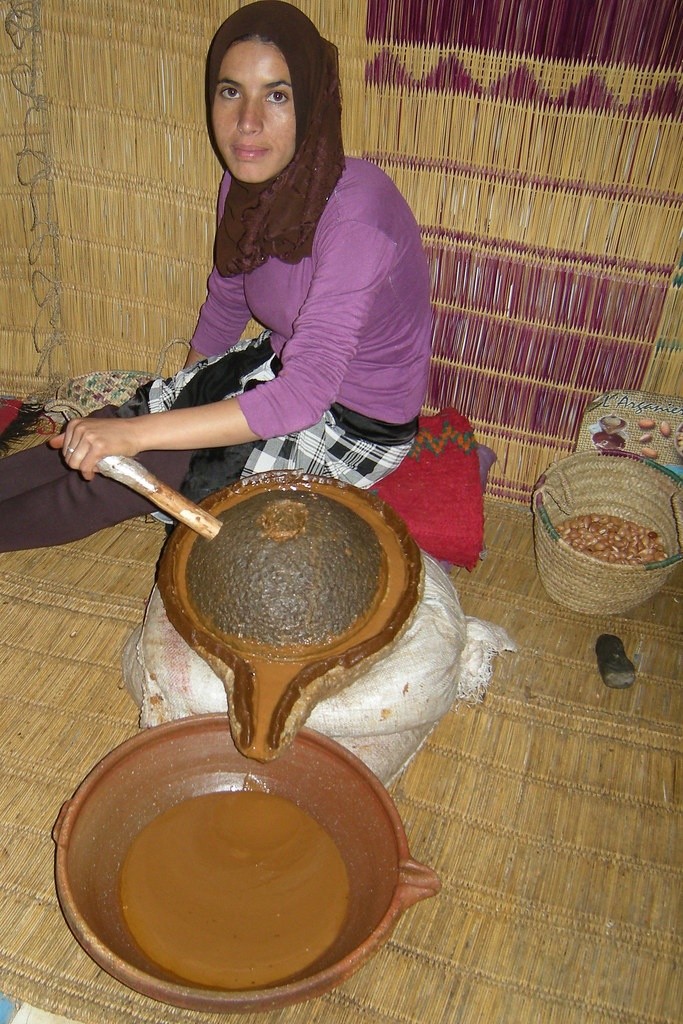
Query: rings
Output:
[[67, 447, 74, 453]]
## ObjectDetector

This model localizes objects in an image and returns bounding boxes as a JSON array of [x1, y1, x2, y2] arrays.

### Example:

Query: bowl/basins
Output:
[[50, 714, 440, 1013]]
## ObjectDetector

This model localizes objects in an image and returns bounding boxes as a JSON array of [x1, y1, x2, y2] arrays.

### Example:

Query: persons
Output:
[[0, 0, 433, 553]]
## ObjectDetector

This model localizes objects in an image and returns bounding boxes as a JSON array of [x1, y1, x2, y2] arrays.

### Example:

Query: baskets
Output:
[[45, 338, 191, 422], [532, 449, 683, 616]]
